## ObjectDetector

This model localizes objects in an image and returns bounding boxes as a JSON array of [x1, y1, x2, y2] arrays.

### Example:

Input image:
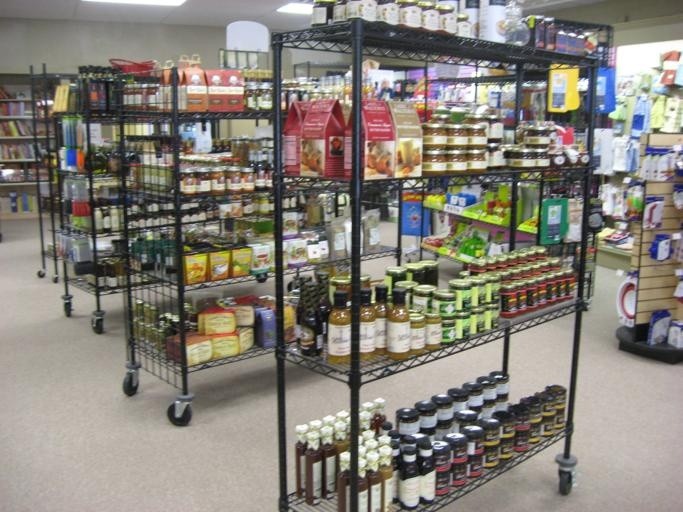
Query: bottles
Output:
[[296, 398, 436, 512], [294, 270, 410, 364], [93, 203, 207, 234], [69, 65, 160, 114]]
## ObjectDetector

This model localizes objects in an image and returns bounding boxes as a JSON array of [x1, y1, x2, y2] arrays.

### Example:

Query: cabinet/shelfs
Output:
[[54, 75, 285, 334], [0, 73, 79, 220], [114, 68, 403, 426], [270, 18, 597, 511], [619, 132, 683, 367], [29, 63, 166, 282]]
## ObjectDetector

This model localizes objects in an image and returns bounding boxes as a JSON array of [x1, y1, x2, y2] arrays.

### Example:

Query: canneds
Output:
[[397, 369, 568, 499], [422, 96, 593, 170], [134, 136, 276, 193], [311, 0, 472, 38], [379, 237, 578, 345]]
